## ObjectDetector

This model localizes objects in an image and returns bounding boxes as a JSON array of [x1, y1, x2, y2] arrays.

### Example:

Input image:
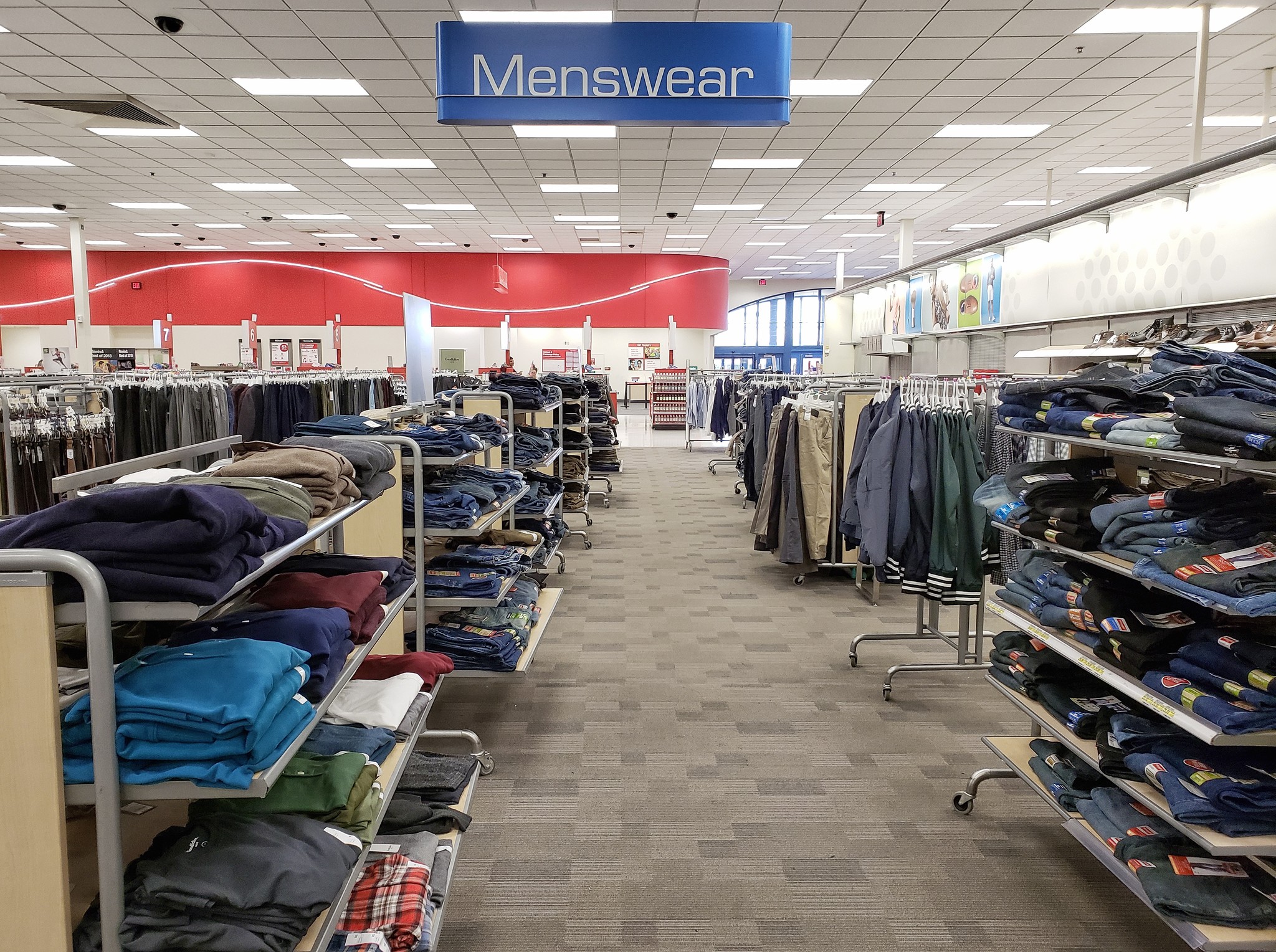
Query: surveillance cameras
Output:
[[172, 224, 179, 226], [155, 16, 184, 34], [667, 213, 678, 219], [319, 243, 326, 246], [198, 237, 206, 241], [392, 235, 401, 239], [464, 244, 470, 247], [371, 238, 378, 241], [261, 217, 272, 222], [174, 243, 181, 246], [628, 245, 635, 248], [53, 204, 67, 210], [16, 241, 24, 245], [521, 239, 528, 243]]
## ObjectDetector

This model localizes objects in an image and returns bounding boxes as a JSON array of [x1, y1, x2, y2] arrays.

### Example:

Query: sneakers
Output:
[[1084, 330, 1114, 348], [1178, 327, 1222, 345], [1218, 324, 1238, 343], [1238, 325, 1268, 345], [992, 316, 995, 321], [1233, 323, 1268, 342], [1236, 321, 1254, 336], [1098, 315, 1175, 346], [988, 317, 990, 322], [1161, 328, 1197, 344], [1246, 324, 1276, 347]]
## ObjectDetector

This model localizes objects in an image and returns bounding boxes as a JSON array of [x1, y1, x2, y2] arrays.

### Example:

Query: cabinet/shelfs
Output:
[[981, 346, 1276, 952], [0, 383, 623, 952], [649, 368, 692, 430]]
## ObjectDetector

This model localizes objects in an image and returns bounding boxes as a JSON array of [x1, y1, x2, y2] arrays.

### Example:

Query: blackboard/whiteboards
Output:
[[403, 292, 435, 406]]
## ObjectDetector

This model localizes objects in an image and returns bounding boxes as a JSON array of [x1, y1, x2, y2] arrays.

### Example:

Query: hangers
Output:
[[0, 369, 406, 399], [690, 371, 1012, 431]]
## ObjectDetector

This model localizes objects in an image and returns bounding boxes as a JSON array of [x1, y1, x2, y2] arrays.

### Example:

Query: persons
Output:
[[98, 364, 112, 373], [52, 348, 67, 368], [96, 361, 104, 373], [635, 360, 642, 370], [890, 284, 901, 334], [987, 258, 996, 322], [929, 271, 950, 331], [510, 357, 517, 374], [629, 359, 637, 370]]
